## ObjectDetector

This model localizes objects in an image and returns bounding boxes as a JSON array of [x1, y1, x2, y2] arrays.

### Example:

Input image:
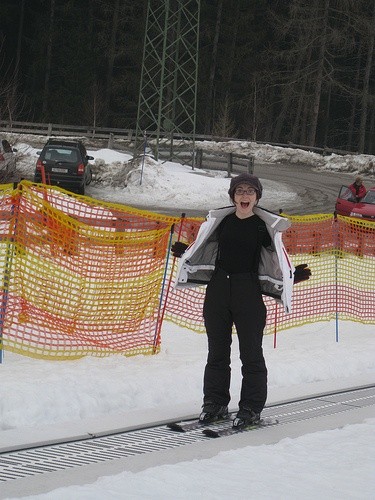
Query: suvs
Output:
[[0, 139, 17, 180]]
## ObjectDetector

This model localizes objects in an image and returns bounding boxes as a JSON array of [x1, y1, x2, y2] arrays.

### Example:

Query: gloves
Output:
[[293, 264, 311, 284], [171, 242, 189, 257]]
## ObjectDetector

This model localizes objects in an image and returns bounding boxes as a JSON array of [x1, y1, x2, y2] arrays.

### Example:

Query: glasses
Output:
[[235, 187, 256, 195]]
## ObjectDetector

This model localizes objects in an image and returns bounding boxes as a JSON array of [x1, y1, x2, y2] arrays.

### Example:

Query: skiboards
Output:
[[167, 415, 235, 432], [202, 419, 279, 437]]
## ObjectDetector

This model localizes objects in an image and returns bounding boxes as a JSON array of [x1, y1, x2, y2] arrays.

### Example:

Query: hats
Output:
[[228, 174, 262, 200], [355, 177, 362, 185]]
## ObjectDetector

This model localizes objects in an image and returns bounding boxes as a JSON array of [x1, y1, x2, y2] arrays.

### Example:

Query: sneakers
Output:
[[233, 410, 259, 426], [199, 407, 228, 421]]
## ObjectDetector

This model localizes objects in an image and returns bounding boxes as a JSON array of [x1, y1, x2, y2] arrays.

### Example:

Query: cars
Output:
[[33, 137, 94, 195], [333, 183, 374, 240]]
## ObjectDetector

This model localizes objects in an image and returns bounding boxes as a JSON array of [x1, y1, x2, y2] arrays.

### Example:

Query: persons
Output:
[[340, 177, 366, 203], [171, 174, 312, 428]]
